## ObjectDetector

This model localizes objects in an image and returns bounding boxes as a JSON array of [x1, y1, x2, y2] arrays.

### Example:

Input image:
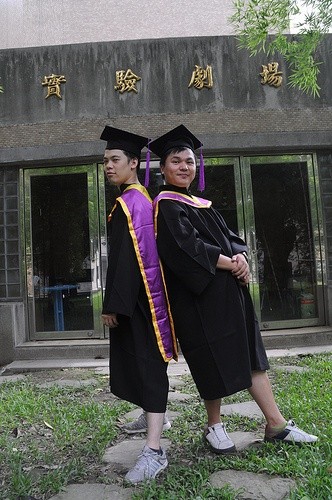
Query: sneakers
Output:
[[264, 420, 320, 447], [122, 412, 171, 433], [204, 423, 235, 453], [123, 445, 168, 487]]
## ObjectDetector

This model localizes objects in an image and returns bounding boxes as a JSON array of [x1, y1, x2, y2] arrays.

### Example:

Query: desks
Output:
[[35, 285, 81, 331]]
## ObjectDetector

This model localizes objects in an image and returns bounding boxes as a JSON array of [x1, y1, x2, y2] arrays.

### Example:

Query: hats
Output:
[[100, 126, 151, 157], [146, 124, 203, 159]]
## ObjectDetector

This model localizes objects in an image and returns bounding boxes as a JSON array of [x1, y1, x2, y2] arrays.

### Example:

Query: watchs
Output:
[[239, 251, 249, 263]]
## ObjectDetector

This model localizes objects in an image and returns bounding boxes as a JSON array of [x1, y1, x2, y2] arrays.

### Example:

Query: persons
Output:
[[145, 123, 321, 457], [98, 126, 180, 488]]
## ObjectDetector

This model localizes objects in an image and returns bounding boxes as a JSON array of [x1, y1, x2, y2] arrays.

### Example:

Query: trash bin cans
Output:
[[299, 293, 318, 320]]
